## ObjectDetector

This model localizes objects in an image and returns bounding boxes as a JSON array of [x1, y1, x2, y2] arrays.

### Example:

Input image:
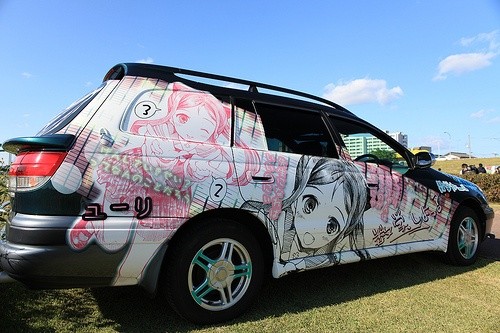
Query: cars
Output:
[[393, 149, 460, 161]]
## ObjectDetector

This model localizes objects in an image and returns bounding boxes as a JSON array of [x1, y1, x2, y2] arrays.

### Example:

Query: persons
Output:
[[462, 163, 486, 176]]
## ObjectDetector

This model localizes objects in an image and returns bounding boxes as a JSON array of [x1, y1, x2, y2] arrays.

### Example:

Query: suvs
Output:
[[0, 63, 496, 326]]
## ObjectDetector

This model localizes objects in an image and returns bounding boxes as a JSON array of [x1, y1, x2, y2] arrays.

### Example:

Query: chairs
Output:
[[298, 142, 322, 156]]
